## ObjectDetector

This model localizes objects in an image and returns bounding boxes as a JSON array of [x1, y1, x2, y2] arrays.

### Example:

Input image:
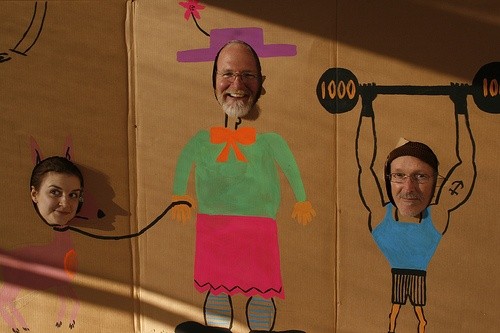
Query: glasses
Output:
[[216, 72, 260, 80], [386, 173, 435, 184]]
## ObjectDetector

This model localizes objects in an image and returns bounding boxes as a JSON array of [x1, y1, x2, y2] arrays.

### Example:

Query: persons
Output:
[[383, 140, 439, 218], [30, 156, 84, 226], [213, 39, 264, 119]]
